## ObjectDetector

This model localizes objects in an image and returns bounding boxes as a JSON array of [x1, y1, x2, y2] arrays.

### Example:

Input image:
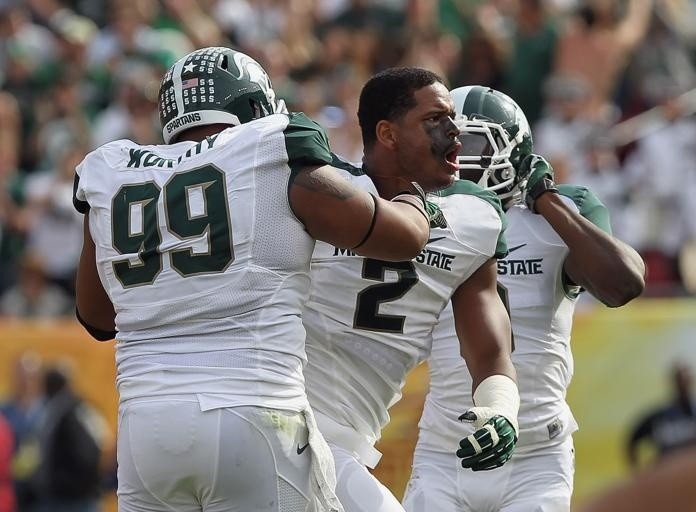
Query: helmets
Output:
[[450, 84, 534, 197], [159, 46, 275, 144]]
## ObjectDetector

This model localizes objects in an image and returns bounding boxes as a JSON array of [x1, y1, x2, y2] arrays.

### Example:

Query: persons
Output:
[[0, 0, 696, 318], [623, 361, 696, 472], [0, 350, 119, 512], [401, 85, 646, 512], [72, 46, 447, 512], [304, 66, 520, 512]]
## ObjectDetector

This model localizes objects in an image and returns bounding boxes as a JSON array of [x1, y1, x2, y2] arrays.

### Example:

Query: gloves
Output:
[[515, 150, 558, 213], [389, 181, 448, 231], [455, 375, 520, 472]]
